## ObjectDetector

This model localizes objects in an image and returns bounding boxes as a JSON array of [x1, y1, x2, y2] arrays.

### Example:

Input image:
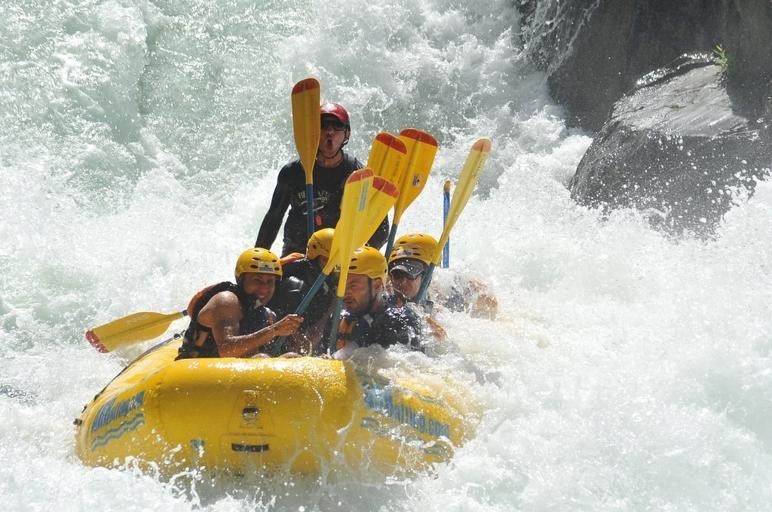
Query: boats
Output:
[[77, 265, 498, 479]]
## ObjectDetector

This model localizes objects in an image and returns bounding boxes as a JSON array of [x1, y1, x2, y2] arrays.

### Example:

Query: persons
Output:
[[267, 227, 339, 357], [388, 247, 434, 306], [174, 248, 304, 361], [254, 101, 389, 251], [317, 246, 436, 355]]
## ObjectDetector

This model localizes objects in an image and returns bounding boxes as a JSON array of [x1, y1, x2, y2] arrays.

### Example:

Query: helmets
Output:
[[333, 246, 387, 292], [388, 244, 430, 269], [306, 228, 335, 260], [320, 102, 350, 141], [235, 247, 283, 297]]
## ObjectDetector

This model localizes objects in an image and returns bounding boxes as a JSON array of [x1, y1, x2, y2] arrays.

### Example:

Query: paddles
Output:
[[416, 140, 489, 301], [383, 128, 438, 260], [330, 169, 374, 359], [270, 176, 400, 358], [290, 78, 320, 238], [87, 311, 188, 354], [365, 133, 405, 183]]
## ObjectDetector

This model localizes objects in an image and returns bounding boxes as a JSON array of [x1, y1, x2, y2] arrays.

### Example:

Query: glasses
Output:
[[321, 120, 346, 131], [392, 271, 423, 281]]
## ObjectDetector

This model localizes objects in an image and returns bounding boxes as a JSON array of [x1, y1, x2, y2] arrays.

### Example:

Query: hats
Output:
[[389, 259, 425, 276]]
